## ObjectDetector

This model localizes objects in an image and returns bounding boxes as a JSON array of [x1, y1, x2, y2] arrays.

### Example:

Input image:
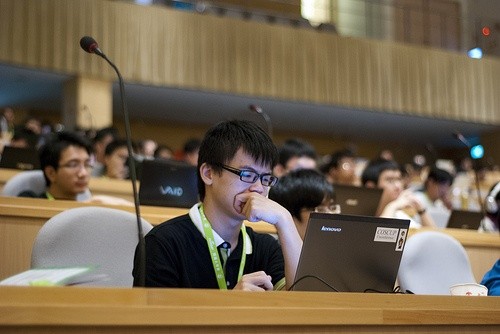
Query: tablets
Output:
[[485, 183, 500, 214]]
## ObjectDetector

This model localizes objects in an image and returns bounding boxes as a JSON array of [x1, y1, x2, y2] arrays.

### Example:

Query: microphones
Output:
[[453, 131, 486, 232], [250, 104, 273, 137], [81, 35, 146, 289]]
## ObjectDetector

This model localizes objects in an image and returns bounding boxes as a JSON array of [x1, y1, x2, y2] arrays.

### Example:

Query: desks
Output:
[[0, 197, 500, 285], [0, 169, 139, 203], [0, 283, 500, 334]]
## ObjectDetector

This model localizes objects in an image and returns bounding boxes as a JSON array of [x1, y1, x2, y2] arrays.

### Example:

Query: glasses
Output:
[[301, 203, 341, 214], [58, 162, 94, 169], [222, 164, 278, 187]]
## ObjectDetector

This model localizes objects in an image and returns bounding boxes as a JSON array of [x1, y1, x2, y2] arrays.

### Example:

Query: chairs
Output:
[[397, 231, 476, 293], [2, 170, 93, 202], [31, 206, 155, 281]]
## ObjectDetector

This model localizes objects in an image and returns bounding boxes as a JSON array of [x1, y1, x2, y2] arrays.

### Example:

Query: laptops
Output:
[[292, 182, 410, 293], [139, 159, 200, 209], [446, 210, 484, 229], [0, 146, 41, 171]]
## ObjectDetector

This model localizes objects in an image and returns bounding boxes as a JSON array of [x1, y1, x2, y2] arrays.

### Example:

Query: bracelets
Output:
[[419, 210, 425, 214]]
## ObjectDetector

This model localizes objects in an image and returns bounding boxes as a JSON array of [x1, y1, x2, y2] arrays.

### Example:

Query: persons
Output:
[[360, 157, 433, 227], [271, 137, 358, 187], [132, 121, 304, 290], [406, 154, 500, 233], [0, 107, 200, 180], [481, 257, 500, 296], [267, 167, 342, 241], [18, 131, 92, 200]]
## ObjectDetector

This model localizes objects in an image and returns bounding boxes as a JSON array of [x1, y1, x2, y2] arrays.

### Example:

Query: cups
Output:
[[449, 283, 489, 297]]
